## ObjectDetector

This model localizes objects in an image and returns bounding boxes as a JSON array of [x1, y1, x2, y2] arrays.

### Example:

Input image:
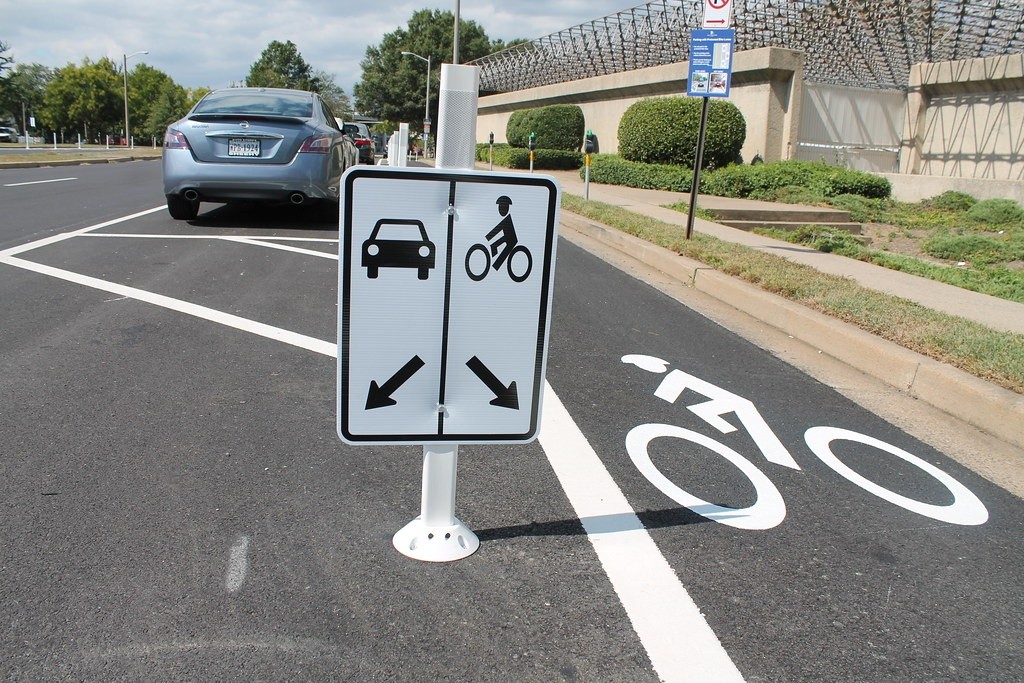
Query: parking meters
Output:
[[528, 132, 537, 172], [489, 131, 494, 169], [584, 129, 600, 201]]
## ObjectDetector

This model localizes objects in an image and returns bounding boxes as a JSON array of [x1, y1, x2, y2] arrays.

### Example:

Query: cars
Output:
[[341, 121, 376, 167], [162, 87, 359, 221], [0, 127, 20, 143]]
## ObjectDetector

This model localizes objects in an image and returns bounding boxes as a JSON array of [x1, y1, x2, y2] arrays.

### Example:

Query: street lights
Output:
[[123, 52, 149, 147], [401, 51, 431, 158]]
